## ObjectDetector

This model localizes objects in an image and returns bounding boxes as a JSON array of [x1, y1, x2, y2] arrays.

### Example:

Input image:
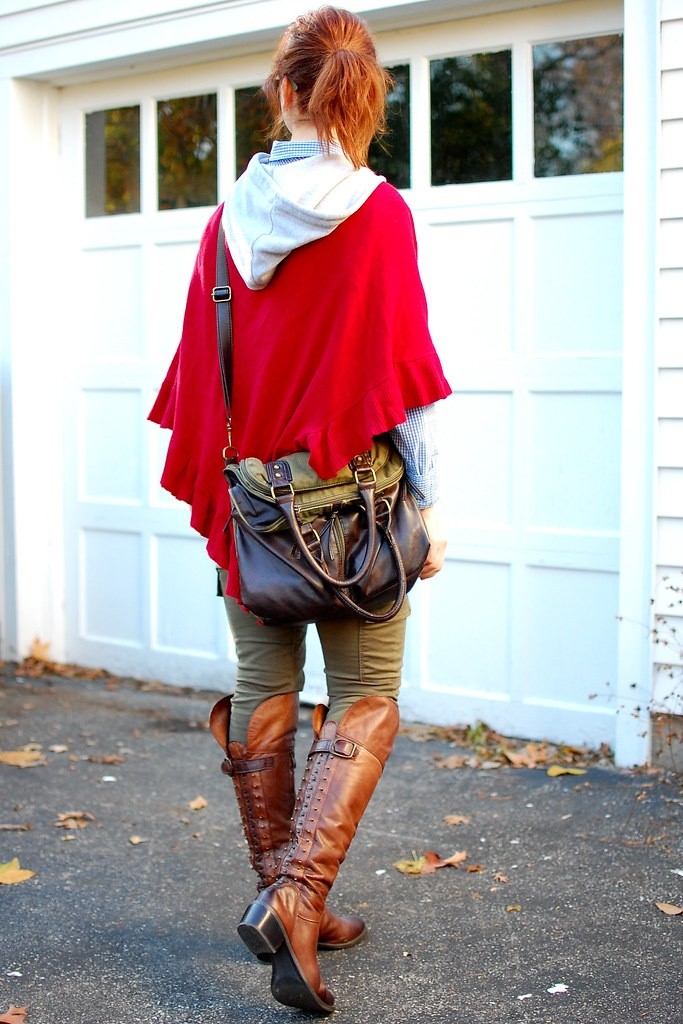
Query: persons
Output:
[[149, 6, 454, 1013]]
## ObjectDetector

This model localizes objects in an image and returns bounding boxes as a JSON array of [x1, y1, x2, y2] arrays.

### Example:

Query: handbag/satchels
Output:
[[220, 430, 431, 628]]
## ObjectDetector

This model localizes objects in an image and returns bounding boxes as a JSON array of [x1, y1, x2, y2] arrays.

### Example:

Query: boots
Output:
[[209, 693, 366, 949], [236, 696, 400, 1013]]
